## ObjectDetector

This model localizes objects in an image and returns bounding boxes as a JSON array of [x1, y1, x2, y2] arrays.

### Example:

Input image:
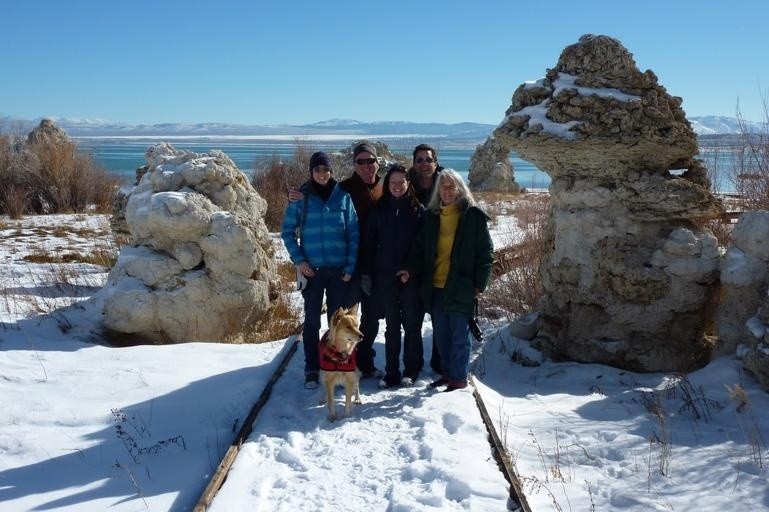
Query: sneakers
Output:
[[401, 367, 419, 387], [443, 384, 466, 392], [303, 372, 319, 390], [377, 370, 402, 389], [361, 366, 387, 380], [426, 377, 448, 389]]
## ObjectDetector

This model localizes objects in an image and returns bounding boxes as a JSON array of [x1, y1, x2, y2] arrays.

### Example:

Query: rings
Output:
[[305, 271, 309, 273]]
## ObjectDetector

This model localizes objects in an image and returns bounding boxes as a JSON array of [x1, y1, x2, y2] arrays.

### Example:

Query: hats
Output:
[[353, 142, 379, 164], [309, 151, 333, 175]]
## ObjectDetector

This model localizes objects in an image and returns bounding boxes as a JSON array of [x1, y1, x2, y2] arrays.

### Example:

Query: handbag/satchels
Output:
[[295, 263, 309, 292]]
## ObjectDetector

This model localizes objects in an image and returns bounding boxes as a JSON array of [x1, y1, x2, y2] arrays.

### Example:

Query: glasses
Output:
[[312, 167, 333, 173], [354, 158, 377, 165], [415, 157, 434, 164]]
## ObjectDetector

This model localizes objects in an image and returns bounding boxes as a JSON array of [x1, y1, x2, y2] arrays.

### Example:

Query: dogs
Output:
[[319, 301, 366, 423]]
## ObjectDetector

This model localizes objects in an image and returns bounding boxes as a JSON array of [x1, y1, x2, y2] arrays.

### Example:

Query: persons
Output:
[[396, 167, 495, 391], [359, 164, 432, 389], [281, 151, 363, 391], [287, 141, 393, 381], [406, 143, 454, 386]]
